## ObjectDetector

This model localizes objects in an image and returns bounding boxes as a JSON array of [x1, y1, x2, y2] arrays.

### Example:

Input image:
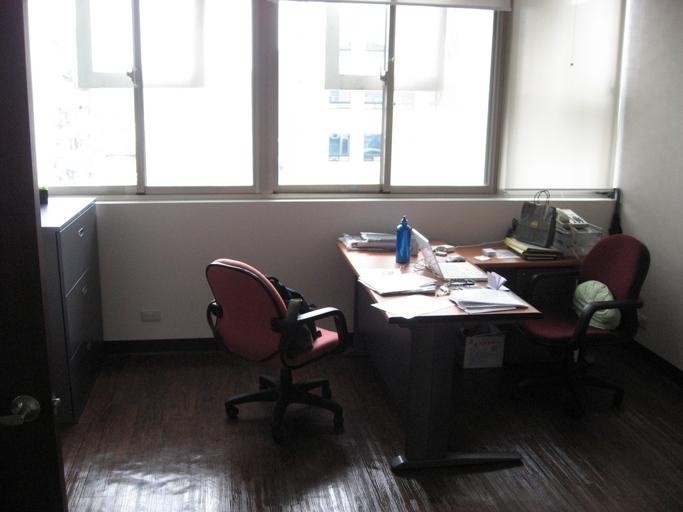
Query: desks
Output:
[[336, 240, 524, 473], [461, 244, 580, 287]]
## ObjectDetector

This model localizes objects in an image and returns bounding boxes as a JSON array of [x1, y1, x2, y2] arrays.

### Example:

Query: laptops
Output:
[[412, 227, 488, 282]]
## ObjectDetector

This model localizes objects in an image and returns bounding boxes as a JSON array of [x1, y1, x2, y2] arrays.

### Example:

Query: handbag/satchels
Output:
[[516, 190, 555, 248]]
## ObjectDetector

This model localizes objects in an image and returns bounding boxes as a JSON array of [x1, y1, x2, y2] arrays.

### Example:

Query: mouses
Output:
[[445, 254, 465, 261]]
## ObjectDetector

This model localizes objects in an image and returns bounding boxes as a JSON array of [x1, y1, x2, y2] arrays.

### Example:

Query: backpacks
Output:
[[267, 276, 321, 341]]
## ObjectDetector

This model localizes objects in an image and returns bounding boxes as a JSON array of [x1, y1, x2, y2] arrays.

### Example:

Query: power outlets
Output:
[[141, 310, 160, 321]]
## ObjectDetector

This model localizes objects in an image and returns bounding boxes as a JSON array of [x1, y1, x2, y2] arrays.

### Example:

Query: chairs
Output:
[[518, 236, 650, 406], [205, 257, 349, 435]]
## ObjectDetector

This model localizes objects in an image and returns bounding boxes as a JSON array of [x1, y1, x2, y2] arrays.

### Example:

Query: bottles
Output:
[[396, 216, 411, 263]]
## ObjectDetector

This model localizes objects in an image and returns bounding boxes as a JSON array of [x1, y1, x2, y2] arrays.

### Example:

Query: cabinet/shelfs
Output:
[[39, 203, 107, 424]]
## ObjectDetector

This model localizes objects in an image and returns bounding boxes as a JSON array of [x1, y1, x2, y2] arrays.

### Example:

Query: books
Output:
[[338, 229, 397, 251], [504, 237, 563, 260]]
